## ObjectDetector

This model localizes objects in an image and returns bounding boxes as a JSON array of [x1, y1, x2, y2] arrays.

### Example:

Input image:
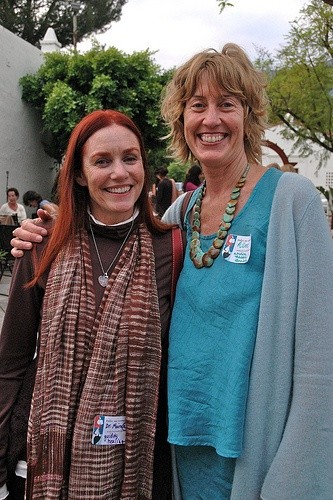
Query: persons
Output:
[[10, 43, 333, 500], [0, 110, 186, 500], [0, 165, 204, 226]]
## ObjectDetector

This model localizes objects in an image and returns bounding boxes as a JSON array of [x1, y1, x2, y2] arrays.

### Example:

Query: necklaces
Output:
[[89, 211, 134, 287], [189, 162, 247, 269]]
